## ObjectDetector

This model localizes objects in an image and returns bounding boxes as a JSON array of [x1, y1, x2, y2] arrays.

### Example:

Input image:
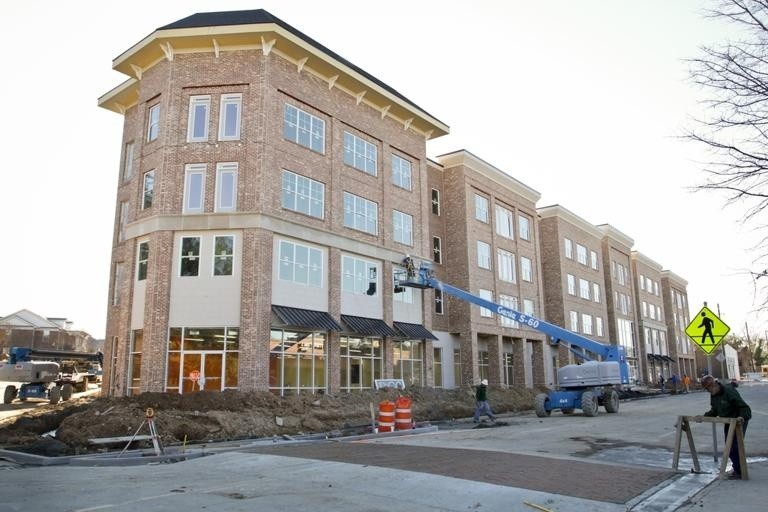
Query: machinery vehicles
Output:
[[393, 259, 630, 417], [1, 346, 103, 405]]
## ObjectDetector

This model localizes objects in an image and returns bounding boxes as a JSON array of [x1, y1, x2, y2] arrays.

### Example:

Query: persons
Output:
[[403, 254, 416, 279], [669, 372, 680, 394], [682, 374, 690, 393], [696, 374, 752, 480], [658, 373, 666, 392], [471, 378, 496, 423]]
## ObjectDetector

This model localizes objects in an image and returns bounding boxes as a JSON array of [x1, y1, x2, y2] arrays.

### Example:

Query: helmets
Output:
[[701, 375, 714, 389], [481, 379, 488, 386]]
